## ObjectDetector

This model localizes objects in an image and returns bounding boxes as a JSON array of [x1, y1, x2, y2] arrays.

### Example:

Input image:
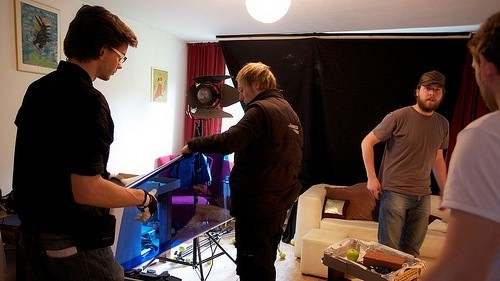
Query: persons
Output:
[[418, 11, 500, 281], [362, 71, 450, 257], [12, 4, 157, 281], [182, 62, 302, 281]]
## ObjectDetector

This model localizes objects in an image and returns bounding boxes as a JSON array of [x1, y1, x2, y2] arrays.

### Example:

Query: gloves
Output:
[[109, 174, 130, 188], [135, 188, 158, 224]]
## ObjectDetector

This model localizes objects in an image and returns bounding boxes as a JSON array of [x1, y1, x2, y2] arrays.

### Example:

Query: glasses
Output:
[[111, 46, 127, 64]]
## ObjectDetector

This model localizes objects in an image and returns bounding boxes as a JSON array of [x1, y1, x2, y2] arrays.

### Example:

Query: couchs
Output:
[[158, 153, 214, 224], [293, 184, 452, 278]]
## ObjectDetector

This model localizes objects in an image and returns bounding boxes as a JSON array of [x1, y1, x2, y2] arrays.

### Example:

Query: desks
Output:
[[0, 173, 181, 281]]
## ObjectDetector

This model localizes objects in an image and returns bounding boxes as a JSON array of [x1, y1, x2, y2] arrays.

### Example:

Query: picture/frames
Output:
[[151, 66, 169, 104], [14, 0, 62, 75]]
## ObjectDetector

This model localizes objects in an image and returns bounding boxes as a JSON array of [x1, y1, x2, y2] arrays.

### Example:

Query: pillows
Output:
[[322, 182, 377, 221]]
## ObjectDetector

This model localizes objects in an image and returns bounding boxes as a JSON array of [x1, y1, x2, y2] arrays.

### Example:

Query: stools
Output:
[[301, 228, 349, 279]]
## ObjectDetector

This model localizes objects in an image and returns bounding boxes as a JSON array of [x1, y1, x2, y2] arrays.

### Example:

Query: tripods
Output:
[[143, 119, 238, 281]]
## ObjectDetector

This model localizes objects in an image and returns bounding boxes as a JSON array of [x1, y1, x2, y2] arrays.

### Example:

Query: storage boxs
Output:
[[363, 252, 406, 271]]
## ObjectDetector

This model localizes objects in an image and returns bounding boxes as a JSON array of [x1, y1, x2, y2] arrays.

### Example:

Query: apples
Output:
[[346, 248, 359, 261]]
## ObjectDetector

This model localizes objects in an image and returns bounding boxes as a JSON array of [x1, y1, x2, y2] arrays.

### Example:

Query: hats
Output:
[[420, 70, 445, 87]]
